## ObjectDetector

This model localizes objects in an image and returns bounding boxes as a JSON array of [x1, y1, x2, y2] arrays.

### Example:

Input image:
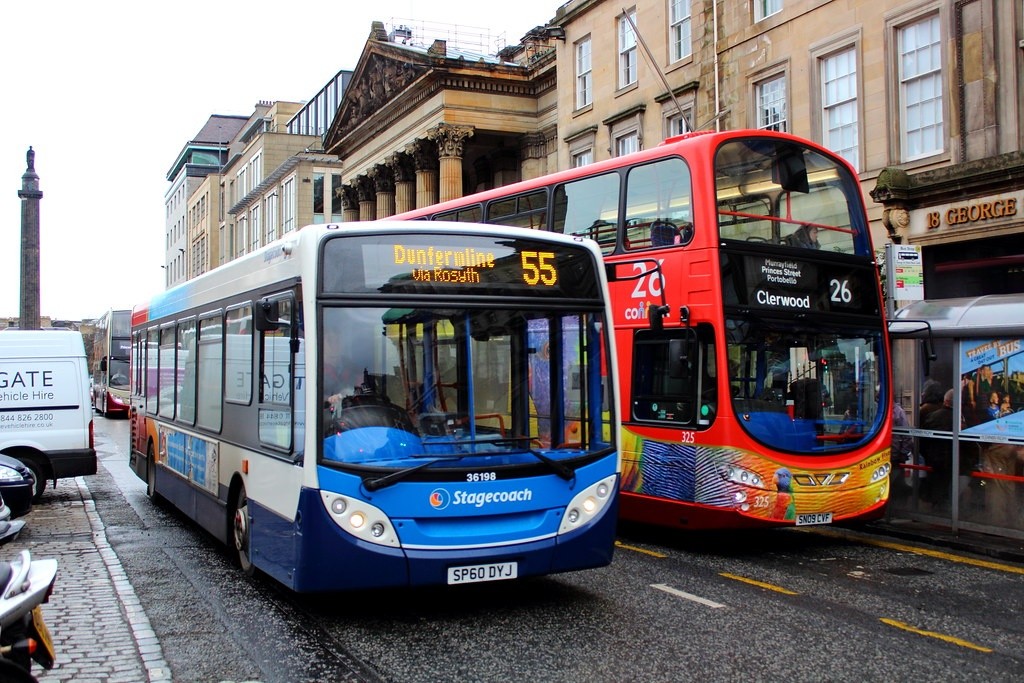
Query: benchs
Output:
[[898, 462, 1024, 483]]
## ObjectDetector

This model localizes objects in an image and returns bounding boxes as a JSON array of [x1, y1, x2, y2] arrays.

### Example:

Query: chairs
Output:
[[746, 236, 787, 245], [650, 221, 694, 247]]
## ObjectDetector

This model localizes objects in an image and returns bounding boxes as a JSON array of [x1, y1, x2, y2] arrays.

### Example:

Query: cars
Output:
[[0, 453, 38, 520]]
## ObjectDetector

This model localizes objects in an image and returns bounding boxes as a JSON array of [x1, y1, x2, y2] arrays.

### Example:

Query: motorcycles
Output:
[[0, 549, 58, 683]]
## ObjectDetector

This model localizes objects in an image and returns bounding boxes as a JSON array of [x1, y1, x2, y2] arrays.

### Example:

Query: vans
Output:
[[179, 336, 305, 454], [0, 330, 98, 505]]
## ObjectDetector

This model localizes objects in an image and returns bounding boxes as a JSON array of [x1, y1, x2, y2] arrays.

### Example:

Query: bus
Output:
[[127, 218, 671, 597], [181, 312, 293, 348], [128, 347, 189, 421], [367, 130, 938, 533], [92, 308, 132, 419]]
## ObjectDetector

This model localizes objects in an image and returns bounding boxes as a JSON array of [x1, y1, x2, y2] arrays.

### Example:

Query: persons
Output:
[[324, 327, 366, 404], [961, 364, 1024, 426], [786, 224, 821, 249], [917, 378, 954, 498], [691, 358, 716, 402], [357, 54, 414, 113], [729, 359, 739, 397], [874, 382, 912, 486], [837, 385, 860, 431], [112, 368, 127, 380], [785, 377, 831, 431], [670, 359, 690, 377]]
